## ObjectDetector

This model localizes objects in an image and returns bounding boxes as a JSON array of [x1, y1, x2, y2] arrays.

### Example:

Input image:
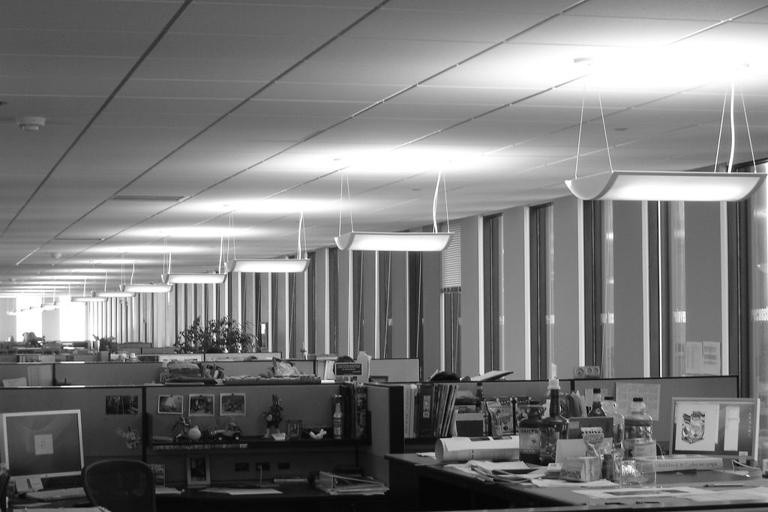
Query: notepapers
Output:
[[16, 476, 43, 490]]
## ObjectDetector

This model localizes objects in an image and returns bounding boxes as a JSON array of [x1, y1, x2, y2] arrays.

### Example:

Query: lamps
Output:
[[9, 210, 227, 315], [333, 171, 454, 251], [562, 68, 766, 202], [223, 211, 310, 273]]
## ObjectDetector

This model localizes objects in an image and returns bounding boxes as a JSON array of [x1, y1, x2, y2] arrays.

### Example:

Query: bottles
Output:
[[333, 402, 345, 439], [476, 381, 489, 435], [587, 388, 623, 453], [519, 400, 543, 464], [539, 385, 569, 466], [623, 397, 654, 461]]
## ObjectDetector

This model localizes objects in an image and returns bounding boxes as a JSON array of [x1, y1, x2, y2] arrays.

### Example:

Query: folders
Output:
[[318, 470, 382, 493]]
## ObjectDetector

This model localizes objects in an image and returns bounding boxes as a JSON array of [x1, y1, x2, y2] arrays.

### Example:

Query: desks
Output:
[[386, 451, 766, 511], [0, 433, 390, 508]]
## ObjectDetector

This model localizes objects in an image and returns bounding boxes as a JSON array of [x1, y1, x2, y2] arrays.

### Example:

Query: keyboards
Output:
[[26, 488, 86, 501]]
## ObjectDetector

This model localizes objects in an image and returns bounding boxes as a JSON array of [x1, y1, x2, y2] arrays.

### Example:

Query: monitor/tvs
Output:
[[0, 408, 84, 483], [669, 396, 761, 460]]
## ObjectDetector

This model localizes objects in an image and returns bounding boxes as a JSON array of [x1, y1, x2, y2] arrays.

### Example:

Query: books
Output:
[[402, 382, 460, 438], [314, 470, 390, 495]]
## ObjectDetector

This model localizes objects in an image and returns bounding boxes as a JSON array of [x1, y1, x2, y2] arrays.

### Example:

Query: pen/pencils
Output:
[[366, 475, 375, 482], [273, 478, 307, 482], [702, 483, 744, 487]]
